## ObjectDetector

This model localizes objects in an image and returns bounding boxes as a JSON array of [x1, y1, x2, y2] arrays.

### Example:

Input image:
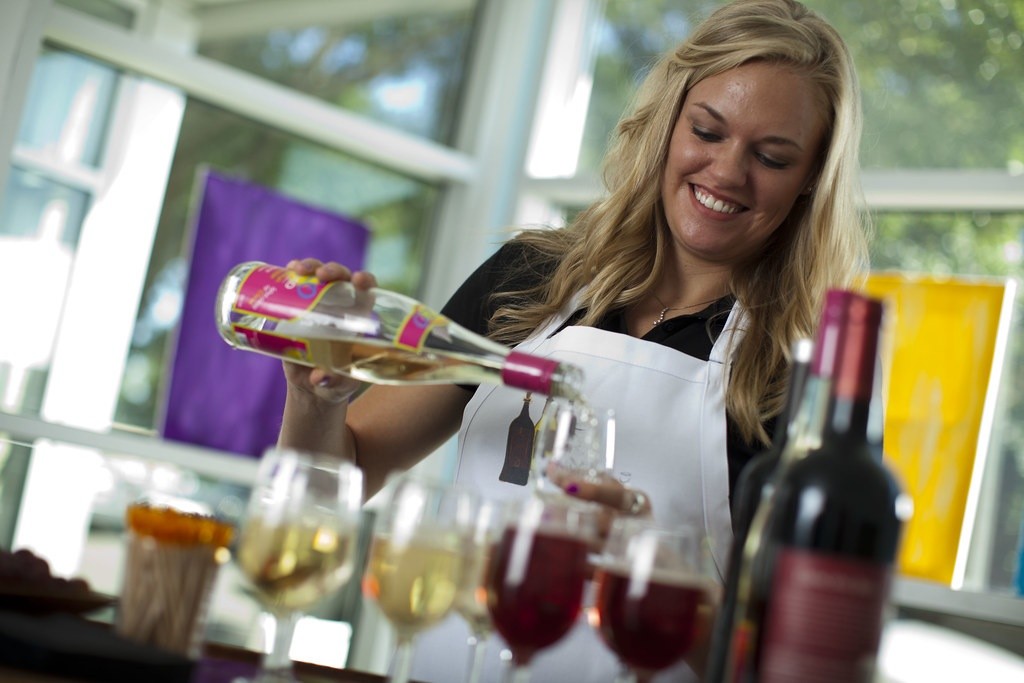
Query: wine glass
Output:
[[482, 483, 592, 683], [452, 494, 519, 682], [233, 445, 363, 683], [365, 473, 465, 682]]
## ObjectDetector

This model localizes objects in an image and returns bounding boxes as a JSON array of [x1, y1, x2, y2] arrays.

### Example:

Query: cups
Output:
[[535, 400, 618, 503], [114, 504, 234, 665], [590, 517, 702, 682]]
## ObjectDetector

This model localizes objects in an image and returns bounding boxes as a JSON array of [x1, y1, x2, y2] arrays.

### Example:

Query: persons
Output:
[[275, 0, 874, 683]]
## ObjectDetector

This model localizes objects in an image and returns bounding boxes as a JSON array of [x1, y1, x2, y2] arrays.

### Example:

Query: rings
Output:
[[627, 493, 645, 515]]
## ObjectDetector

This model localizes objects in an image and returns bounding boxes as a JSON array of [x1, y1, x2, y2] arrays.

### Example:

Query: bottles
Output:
[[705, 290, 905, 682], [214, 259, 584, 401]]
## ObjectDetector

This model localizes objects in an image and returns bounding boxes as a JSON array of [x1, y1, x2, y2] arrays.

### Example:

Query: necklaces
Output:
[[644, 286, 734, 324]]
